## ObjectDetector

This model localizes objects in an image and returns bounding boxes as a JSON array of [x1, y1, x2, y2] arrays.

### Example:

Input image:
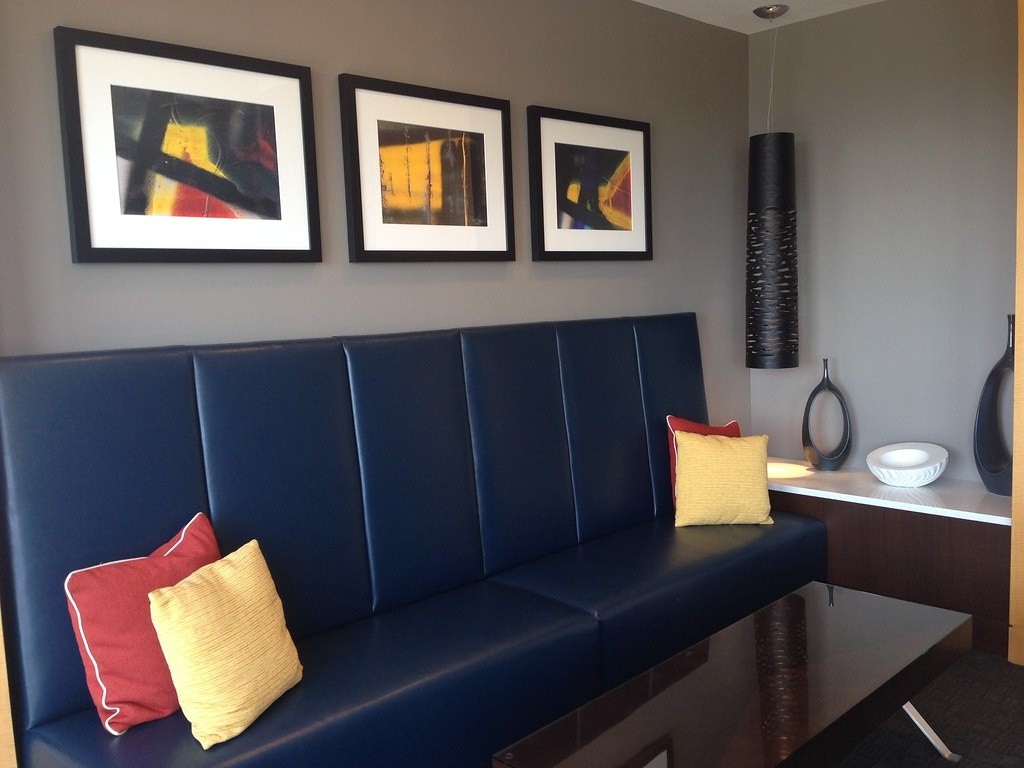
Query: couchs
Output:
[[0, 308, 830, 768]]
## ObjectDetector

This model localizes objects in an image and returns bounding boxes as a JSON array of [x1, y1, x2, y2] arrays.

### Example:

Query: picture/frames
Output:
[[53, 23, 325, 269], [527, 102, 657, 267], [337, 68, 519, 266]]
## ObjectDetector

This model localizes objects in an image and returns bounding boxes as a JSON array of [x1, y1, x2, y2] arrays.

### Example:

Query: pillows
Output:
[[144, 539, 305, 752], [671, 431, 777, 529], [65, 512, 225, 738], [664, 414, 746, 520]]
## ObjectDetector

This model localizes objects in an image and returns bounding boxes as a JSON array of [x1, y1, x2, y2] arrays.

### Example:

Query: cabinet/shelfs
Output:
[[767, 458, 1013, 654]]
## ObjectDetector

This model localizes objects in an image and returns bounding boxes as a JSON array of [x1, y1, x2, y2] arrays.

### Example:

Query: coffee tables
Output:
[[485, 579, 974, 768]]
